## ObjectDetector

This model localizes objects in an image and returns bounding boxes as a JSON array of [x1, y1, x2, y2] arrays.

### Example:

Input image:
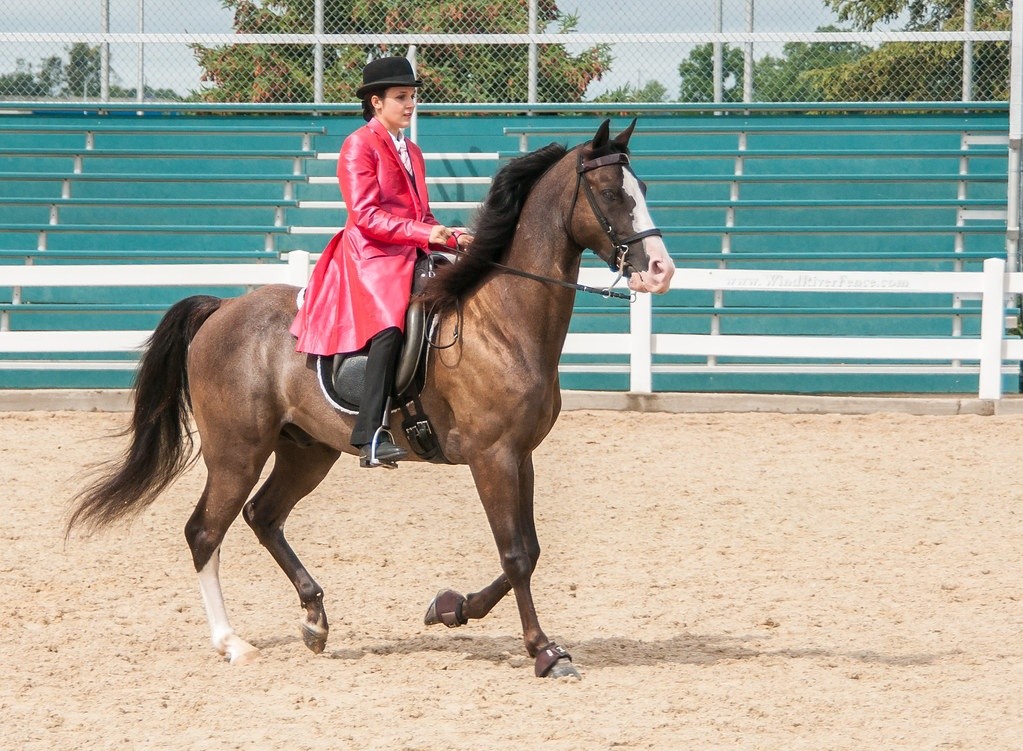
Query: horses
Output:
[[62, 116, 678, 681]]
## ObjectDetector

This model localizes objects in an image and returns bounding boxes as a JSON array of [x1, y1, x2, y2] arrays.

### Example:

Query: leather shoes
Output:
[[358, 441, 407, 468]]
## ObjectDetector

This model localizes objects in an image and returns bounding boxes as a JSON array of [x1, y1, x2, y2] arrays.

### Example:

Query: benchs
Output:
[[0, 120, 329, 323], [492, 123, 1021, 329]]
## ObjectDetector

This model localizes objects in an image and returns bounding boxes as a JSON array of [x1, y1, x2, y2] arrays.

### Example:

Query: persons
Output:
[[292, 56, 475, 469]]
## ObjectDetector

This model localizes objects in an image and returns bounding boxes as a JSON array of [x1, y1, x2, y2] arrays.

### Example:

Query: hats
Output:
[[356, 56, 423, 100]]
